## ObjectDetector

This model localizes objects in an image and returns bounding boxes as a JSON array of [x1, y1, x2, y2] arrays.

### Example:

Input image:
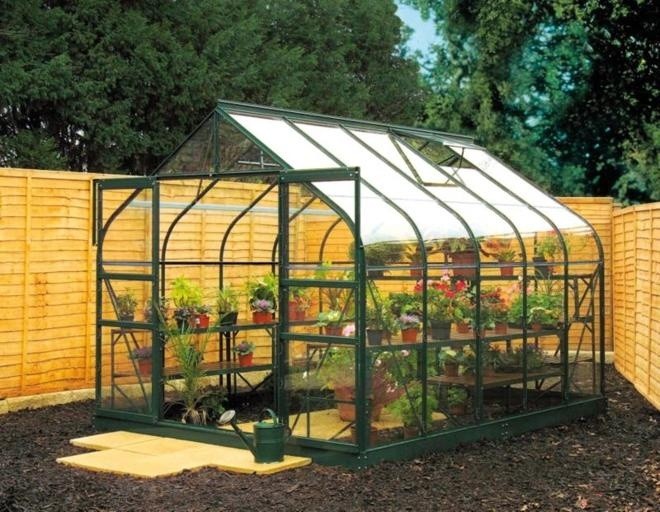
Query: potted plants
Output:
[[114, 287, 138, 326], [170, 275, 213, 332], [212, 285, 241, 330], [155, 307, 232, 427]]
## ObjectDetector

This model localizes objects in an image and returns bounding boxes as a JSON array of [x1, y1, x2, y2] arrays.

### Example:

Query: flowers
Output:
[[130, 344, 157, 378], [145, 296, 170, 328], [287, 278, 312, 321], [232, 340, 256, 369], [243, 273, 280, 324]]
